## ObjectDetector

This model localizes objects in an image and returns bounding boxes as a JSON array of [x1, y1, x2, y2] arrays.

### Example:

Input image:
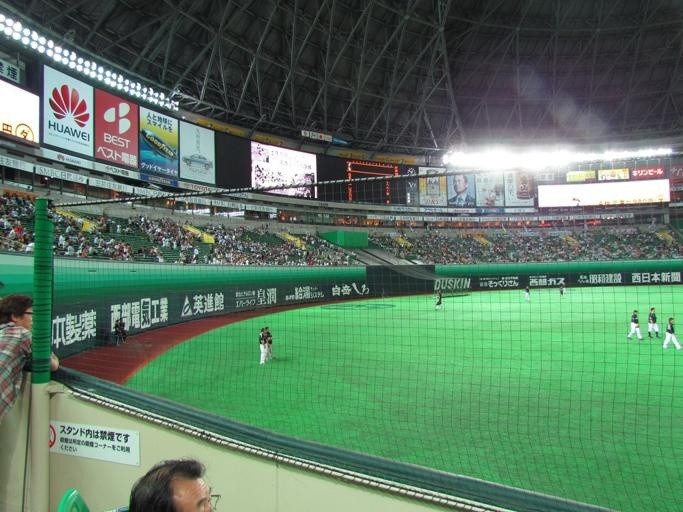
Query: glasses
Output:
[[24, 312, 32, 314], [198, 495, 221, 512]]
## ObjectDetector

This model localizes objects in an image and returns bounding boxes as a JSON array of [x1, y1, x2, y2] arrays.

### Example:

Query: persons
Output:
[[525, 284, 531, 302], [0, 192, 683, 267], [0, 294, 60, 418], [663, 317, 682, 351], [435, 289, 443, 310], [648, 307, 660, 339], [128, 457, 222, 511], [627, 310, 644, 340], [115, 317, 127, 347], [259, 327, 273, 365], [559, 283, 564, 297], [448, 174, 476, 206]]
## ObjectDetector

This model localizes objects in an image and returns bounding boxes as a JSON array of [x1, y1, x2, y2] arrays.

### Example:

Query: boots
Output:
[[648, 332, 660, 338]]
